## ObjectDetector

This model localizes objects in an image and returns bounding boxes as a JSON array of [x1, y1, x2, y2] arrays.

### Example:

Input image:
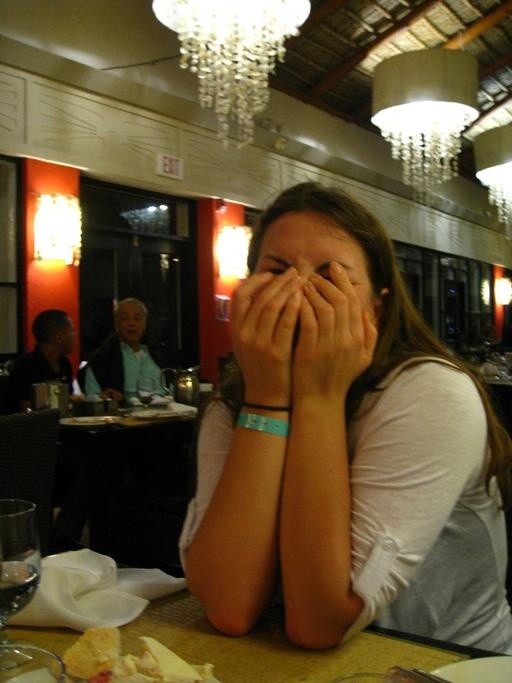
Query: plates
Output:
[[58, 410, 179, 427]]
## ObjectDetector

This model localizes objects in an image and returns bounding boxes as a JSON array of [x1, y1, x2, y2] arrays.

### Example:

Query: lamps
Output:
[[372, 49, 483, 192], [474, 122, 512, 222], [152, 0, 311, 149]]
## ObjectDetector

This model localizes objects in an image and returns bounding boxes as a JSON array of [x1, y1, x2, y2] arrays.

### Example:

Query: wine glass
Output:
[[0, 495, 64, 670], [136, 376, 156, 411]]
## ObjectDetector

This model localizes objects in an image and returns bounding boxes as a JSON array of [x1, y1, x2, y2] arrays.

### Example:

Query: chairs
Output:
[[0, 408, 61, 557]]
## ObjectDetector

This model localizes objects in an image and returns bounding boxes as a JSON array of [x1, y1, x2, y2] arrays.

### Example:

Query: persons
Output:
[[7, 309, 122, 549], [83, 297, 174, 407], [178, 176, 512, 655]]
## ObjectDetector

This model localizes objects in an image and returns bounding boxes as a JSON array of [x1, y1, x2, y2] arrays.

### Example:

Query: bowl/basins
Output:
[[73, 400, 119, 417]]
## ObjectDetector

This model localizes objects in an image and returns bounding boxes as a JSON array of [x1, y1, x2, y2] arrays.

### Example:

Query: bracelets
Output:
[[236, 412, 290, 437], [243, 403, 291, 411]]
